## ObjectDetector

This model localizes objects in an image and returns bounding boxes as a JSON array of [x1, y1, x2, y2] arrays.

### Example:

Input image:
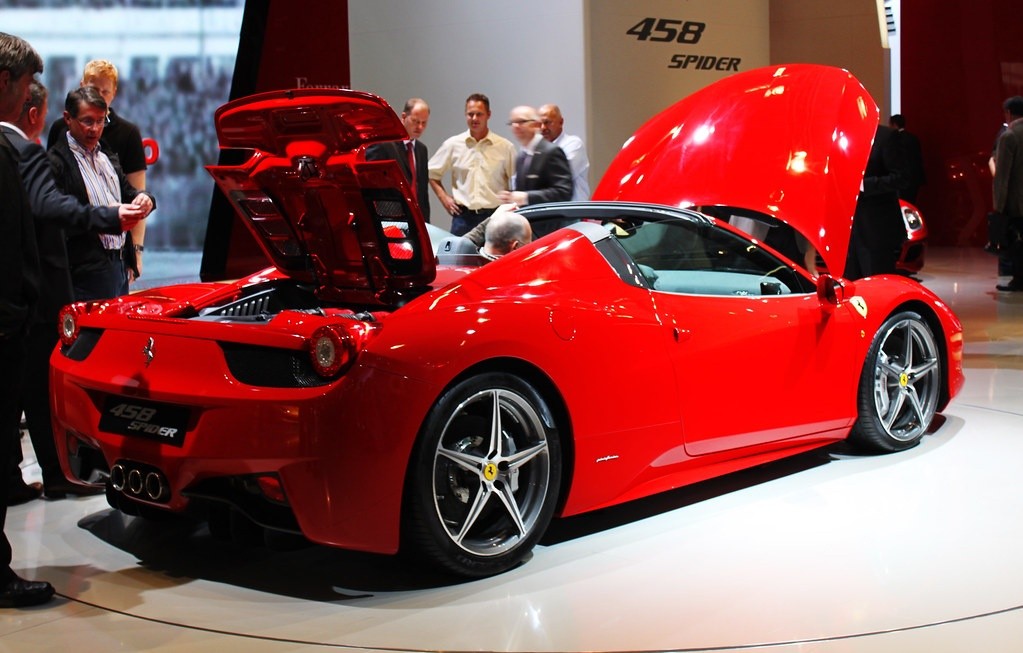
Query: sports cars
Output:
[[47, 63, 963, 581]]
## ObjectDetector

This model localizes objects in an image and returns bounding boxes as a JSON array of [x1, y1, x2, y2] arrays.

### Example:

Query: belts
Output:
[[462, 205, 494, 215], [105, 249, 127, 260]]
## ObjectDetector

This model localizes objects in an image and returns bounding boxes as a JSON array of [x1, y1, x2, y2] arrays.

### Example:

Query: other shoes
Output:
[[7, 482, 43, 505], [43, 483, 103, 497], [0, 576, 55, 608], [996, 279, 1023, 290]]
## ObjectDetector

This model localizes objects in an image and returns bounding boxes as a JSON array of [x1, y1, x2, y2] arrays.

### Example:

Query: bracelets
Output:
[[133, 244, 144, 252]]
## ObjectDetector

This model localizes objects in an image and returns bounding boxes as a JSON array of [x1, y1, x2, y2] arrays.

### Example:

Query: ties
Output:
[[406, 142, 414, 168]]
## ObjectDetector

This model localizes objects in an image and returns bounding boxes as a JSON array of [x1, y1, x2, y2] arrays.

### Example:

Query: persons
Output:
[[497, 105, 572, 242], [367, 97, 430, 224], [0, 31, 156, 609], [989, 96, 1023, 291], [427, 94, 518, 237], [848, 116, 907, 280], [705, 214, 796, 275], [539, 104, 591, 225], [459, 202, 532, 262], [889, 115, 927, 204]]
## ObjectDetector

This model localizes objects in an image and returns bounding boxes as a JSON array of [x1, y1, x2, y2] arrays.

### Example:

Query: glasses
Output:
[[71, 115, 110, 133], [505, 119, 535, 126]]
